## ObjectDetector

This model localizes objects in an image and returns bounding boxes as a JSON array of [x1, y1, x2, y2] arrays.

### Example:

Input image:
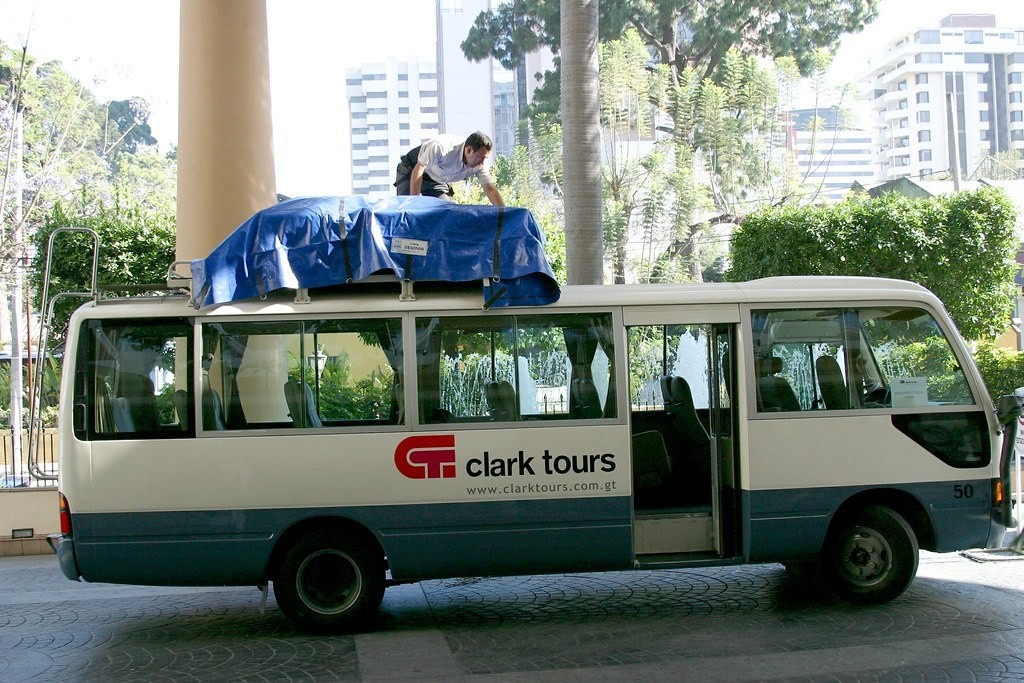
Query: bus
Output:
[[27, 226, 1023, 636]]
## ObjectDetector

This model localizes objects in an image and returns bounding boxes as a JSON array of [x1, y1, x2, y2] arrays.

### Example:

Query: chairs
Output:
[[173, 388, 229, 430], [484, 380, 522, 421], [571, 377, 730, 491], [754, 357, 802, 412], [288, 381, 324, 429], [109, 395, 137, 433], [816, 356, 848, 409]]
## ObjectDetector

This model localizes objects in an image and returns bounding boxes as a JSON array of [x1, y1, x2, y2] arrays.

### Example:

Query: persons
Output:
[[395, 130, 504, 206]]
